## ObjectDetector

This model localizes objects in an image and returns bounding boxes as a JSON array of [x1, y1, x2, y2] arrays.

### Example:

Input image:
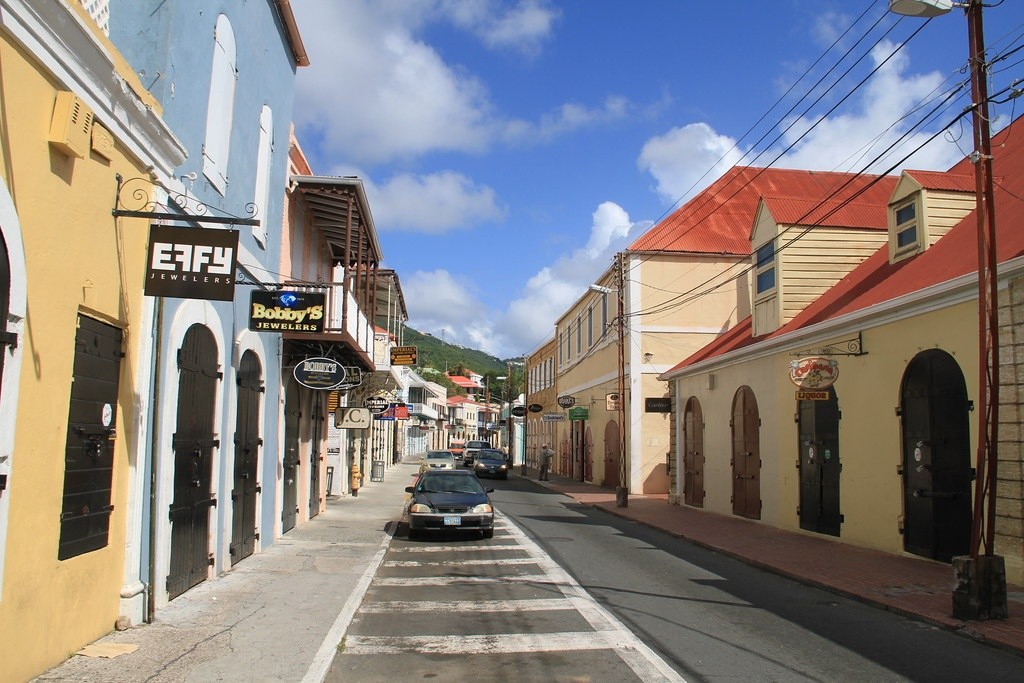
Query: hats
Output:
[[542, 444, 548, 447]]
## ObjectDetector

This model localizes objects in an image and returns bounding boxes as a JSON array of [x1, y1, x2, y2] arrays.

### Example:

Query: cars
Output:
[[472, 449, 511, 479], [418, 450, 459, 476], [405, 469, 495, 538]]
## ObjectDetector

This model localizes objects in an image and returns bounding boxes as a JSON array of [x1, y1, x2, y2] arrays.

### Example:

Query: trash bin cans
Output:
[[372, 460, 385, 482]]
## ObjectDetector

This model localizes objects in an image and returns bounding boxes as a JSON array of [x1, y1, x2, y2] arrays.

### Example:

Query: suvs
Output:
[[462, 440, 493, 467], [448, 441, 467, 458]]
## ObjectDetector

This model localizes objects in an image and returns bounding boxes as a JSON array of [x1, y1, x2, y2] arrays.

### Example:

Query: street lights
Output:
[[588, 285, 631, 508], [881, 0, 1007, 619]]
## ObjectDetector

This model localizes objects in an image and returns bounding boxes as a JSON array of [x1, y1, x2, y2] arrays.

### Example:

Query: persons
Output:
[[502, 441, 510, 462], [539, 443, 556, 481]]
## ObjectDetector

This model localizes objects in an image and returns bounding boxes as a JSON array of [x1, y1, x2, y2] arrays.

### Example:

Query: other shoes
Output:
[[539, 479, 543, 481], [544, 479, 549, 481]]
